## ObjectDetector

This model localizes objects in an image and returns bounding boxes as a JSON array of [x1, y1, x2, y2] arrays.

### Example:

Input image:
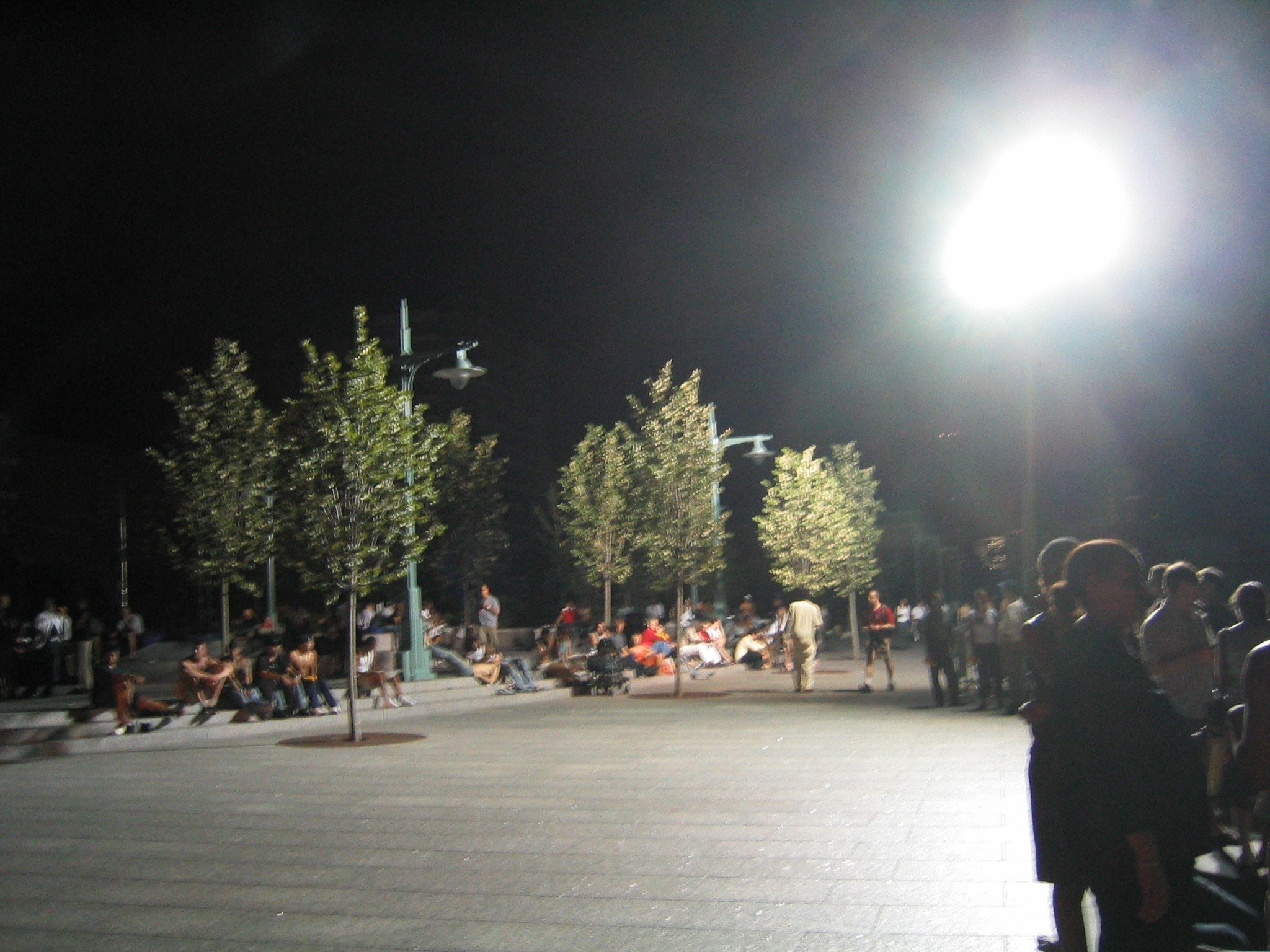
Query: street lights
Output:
[[394, 298, 490, 681], [709, 402, 778, 626]]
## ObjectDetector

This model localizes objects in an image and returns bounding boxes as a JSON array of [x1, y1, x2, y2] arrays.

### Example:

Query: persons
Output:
[[1050, 539, 1213, 952], [0, 583, 793, 735], [1021, 536, 1090, 952], [782, 588, 823, 692], [858, 589, 895, 692], [892, 561, 1270, 872]]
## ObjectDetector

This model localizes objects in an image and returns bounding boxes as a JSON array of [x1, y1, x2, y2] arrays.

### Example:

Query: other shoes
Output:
[[111, 694, 412, 736], [887, 681, 894, 691], [857, 683, 870, 693]]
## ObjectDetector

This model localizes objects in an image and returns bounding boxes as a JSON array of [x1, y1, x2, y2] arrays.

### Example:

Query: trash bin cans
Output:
[[59, 640, 91, 689]]
